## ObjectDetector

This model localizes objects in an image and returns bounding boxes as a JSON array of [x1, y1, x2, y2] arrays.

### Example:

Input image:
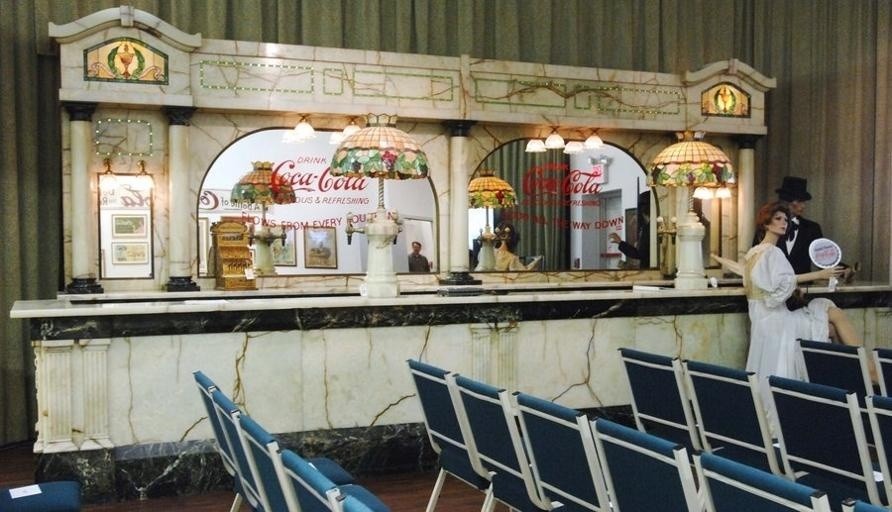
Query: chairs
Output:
[[0, 337, 892, 512]]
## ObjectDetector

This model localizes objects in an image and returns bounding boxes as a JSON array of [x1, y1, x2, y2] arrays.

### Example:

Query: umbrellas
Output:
[[712, 253, 743, 278]]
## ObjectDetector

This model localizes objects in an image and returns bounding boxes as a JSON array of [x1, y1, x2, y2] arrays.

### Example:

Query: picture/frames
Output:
[[267, 224, 297, 268], [302, 223, 337, 268]]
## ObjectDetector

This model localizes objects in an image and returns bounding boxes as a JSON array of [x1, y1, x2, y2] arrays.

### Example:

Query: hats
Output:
[[776, 176, 811, 200]]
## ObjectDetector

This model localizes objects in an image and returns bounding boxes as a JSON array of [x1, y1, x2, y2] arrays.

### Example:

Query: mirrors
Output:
[[653, 180, 722, 271], [468, 139, 658, 270], [196, 126, 439, 275]]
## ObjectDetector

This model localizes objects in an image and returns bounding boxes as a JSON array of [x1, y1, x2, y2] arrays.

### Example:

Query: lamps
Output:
[[98, 161, 154, 192], [644, 130, 734, 289], [524, 138, 583, 154], [715, 179, 730, 201], [589, 155, 612, 184], [343, 116, 361, 135], [291, 113, 318, 141], [328, 112, 430, 299], [470, 169, 521, 269], [234, 163, 301, 276], [584, 126, 603, 150], [545, 126, 565, 150]]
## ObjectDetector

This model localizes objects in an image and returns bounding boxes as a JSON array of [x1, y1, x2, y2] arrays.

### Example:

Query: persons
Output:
[[608, 190, 649, 269], [407, 242, 431, 273], [752, 176, 856, 312], [741, 201, 860, 382]]
